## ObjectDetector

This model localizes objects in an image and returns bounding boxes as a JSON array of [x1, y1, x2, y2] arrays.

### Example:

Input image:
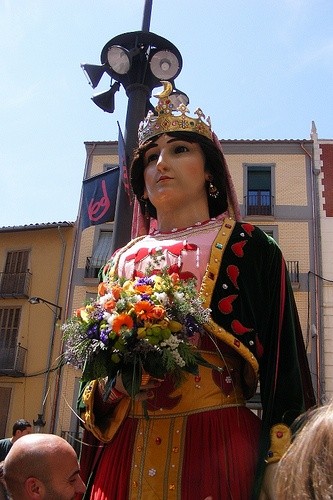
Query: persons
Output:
[[77, 81, 317, 500], [0, 402, 332, 500]]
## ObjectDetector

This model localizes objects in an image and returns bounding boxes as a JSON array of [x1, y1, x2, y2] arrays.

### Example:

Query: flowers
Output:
[[56, 245, 216, 422]]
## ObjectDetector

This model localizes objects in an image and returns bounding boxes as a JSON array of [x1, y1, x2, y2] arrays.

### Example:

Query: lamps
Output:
[[29, 296, 62, 319]]
